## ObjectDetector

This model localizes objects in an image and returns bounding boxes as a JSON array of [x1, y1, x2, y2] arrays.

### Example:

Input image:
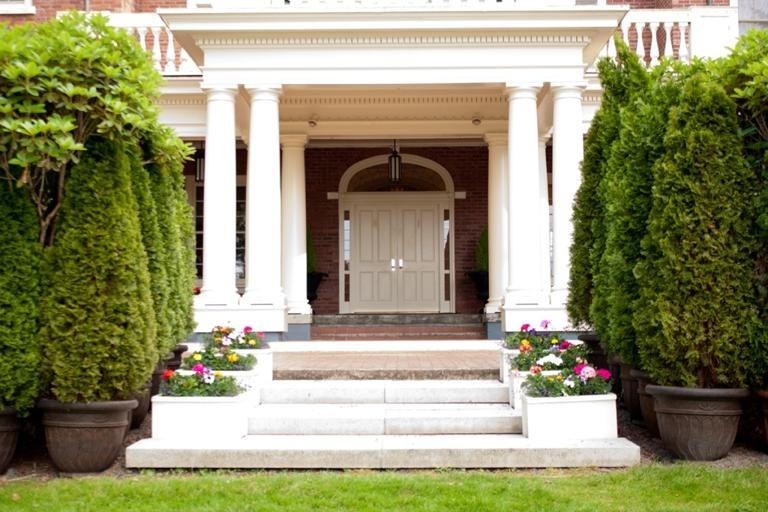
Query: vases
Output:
[[147, 347, 271, 444], [496, 346, 620, 441]]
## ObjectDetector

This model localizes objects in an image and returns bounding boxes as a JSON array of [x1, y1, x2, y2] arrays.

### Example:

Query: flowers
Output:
[[157, 327, 271, 398], [501, 319, 613, 395]]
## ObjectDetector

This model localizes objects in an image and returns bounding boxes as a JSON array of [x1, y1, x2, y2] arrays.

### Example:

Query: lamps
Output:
[[387, 139, 403, 183]]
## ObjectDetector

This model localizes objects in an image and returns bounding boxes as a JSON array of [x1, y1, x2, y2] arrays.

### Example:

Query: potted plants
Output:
[[0, 134, 197, 474], [573, 74, 765, 463]]
